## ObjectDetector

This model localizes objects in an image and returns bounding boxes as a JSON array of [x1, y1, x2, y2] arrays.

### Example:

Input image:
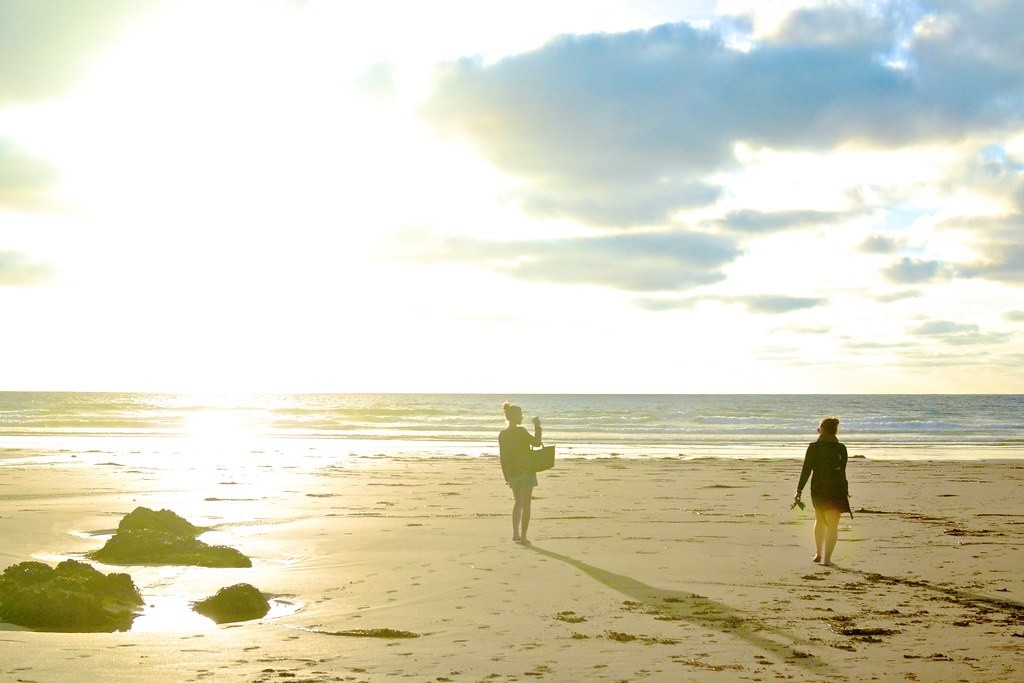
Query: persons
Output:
[[498, 402, 542, 544], [794, 418, 848, 566]]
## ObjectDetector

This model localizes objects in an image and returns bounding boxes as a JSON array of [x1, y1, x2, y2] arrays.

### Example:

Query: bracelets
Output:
[[796, 488, 802, 493]]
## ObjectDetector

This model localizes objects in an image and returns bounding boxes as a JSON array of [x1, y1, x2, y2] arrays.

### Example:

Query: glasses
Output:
[[817, 427, 821, 434]]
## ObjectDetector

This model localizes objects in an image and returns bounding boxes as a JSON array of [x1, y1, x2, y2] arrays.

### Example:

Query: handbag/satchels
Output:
[[834, 466, 848, 497], [530, 442, 554, 472]]
